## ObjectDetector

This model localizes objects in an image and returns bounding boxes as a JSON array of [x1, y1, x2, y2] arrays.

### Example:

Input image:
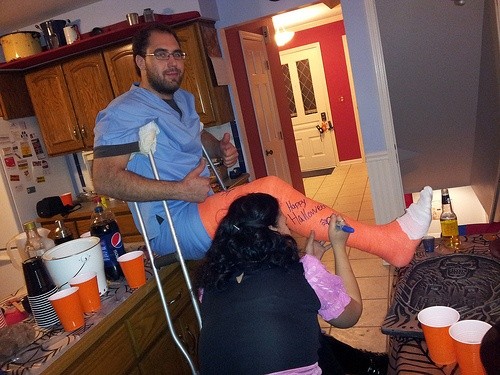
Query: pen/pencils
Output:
[[336, 223, 354, 233]]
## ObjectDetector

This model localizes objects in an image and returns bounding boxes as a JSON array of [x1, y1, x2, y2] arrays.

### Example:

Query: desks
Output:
[[386, 221, 500, 375]]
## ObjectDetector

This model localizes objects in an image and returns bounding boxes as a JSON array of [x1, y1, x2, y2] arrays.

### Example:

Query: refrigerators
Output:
[[0, 116, 85, 303]]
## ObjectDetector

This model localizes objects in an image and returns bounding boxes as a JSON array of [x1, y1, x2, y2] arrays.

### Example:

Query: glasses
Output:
[[144, 50, 186, 60]]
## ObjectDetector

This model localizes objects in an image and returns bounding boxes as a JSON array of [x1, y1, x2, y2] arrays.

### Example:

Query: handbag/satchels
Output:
[[37, 196, 82, 218]]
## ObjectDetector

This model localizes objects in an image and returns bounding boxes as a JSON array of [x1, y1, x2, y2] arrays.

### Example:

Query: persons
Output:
[[91, 24, 433, 269], [192, 192, 363, 375]]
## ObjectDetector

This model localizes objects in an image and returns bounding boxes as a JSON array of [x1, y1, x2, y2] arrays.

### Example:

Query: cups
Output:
[[125, 13, 139, 25], [70, 271, 102, 314], [21, 255, 61, 327], [117, 250, 146, 288], [448, 319, 493, 375], [61, 192, 73, 206], [63, 26, 78, 45], [418, 305, 461, 365], [423, 236, 435, 253], [47, 286, 86, 332]]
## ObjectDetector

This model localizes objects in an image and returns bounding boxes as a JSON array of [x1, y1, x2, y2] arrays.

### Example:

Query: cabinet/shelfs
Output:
[[24, 49, 114, 157], [36, 180, 262, 243], [0, 246, 204, 375], [103, 17, 235, 128]]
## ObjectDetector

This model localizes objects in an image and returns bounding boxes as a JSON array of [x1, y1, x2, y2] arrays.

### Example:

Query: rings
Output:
[[337, 220, 344, 222]]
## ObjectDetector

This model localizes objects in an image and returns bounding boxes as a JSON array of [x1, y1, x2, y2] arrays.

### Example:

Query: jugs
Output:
[[5, 226, 55, 271]]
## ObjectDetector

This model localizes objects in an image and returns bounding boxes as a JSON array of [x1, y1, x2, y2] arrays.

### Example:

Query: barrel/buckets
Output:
[[0, 30, 43, 63], [42, 236, 109, 296], [39, 18, 67, 50]]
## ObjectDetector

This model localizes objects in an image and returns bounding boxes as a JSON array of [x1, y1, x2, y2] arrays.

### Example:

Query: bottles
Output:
[[90, 206, 127, 282], [440, 187, 460, 249], [52, 219, 75, 246], [143, 7, 156, 22], [92, 196, 118, 223], [21, 221, 47, 259]]
